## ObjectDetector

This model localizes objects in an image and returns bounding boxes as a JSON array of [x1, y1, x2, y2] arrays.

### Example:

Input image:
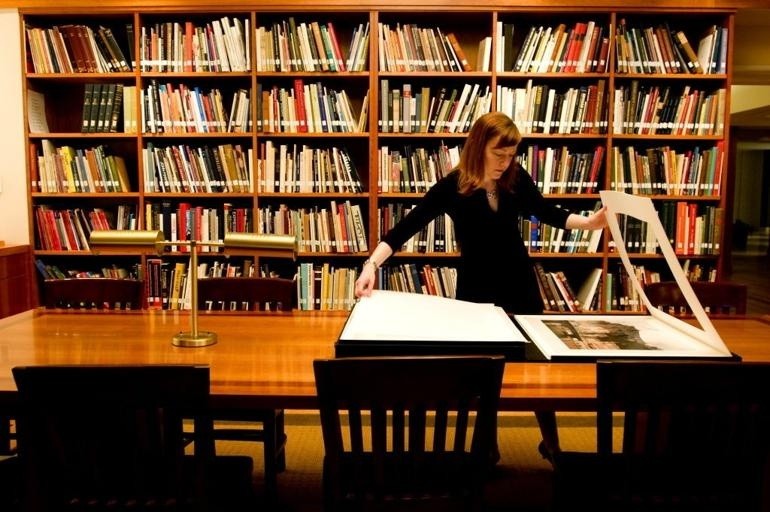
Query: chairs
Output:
[[197, 276, 299, 310], [311, 353, 505, 510], [194, 407, 288, 480], [642, 281, 749, 315], [555, 361, 769, 510], [46, 278, 144, 315], [13, 362, 253, 512]]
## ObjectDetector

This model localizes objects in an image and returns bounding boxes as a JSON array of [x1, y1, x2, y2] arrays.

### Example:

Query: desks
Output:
[[0, 276, 770, 479]]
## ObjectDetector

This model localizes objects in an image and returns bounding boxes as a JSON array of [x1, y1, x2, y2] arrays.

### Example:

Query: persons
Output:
[[354, 110, 610, 479]]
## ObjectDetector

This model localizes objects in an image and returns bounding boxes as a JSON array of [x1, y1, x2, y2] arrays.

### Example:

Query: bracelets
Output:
[[364, 260, 377, 273]]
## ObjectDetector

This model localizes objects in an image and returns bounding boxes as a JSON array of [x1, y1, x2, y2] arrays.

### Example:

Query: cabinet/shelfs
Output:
[[20, 4, 375, 309], [374, 3, 740, 310]]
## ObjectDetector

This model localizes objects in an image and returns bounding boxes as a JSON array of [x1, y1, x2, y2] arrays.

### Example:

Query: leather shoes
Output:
[[538, 439, 564, 469]]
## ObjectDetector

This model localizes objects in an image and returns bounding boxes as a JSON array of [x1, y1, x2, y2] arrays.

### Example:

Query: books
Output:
[[139, 16, 256, 311], [498, 20, 608, 313], [615, 16, 727, 312], [22, 17, 139, 311], [257, 18, 370, 312], [378, 21, 493, 298]]
